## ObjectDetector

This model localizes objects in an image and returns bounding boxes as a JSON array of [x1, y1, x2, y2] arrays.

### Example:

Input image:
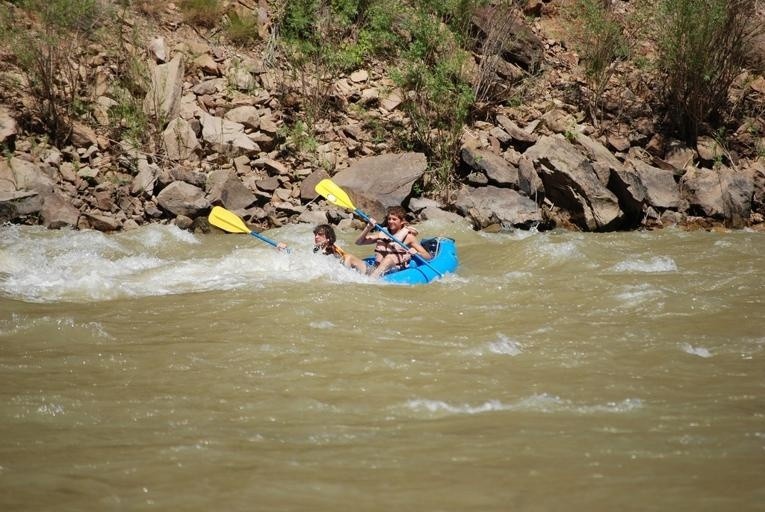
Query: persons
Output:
[[341, 205, 432, 280], [276, 222, 343, 259]]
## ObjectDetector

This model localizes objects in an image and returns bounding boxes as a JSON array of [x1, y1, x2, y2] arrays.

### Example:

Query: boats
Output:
[[361, 236, 459, 286]]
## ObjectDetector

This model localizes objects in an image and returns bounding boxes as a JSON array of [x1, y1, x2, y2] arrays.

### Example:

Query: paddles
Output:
[[208, 205, 292, 254], [315, 178, 444, 279]]
